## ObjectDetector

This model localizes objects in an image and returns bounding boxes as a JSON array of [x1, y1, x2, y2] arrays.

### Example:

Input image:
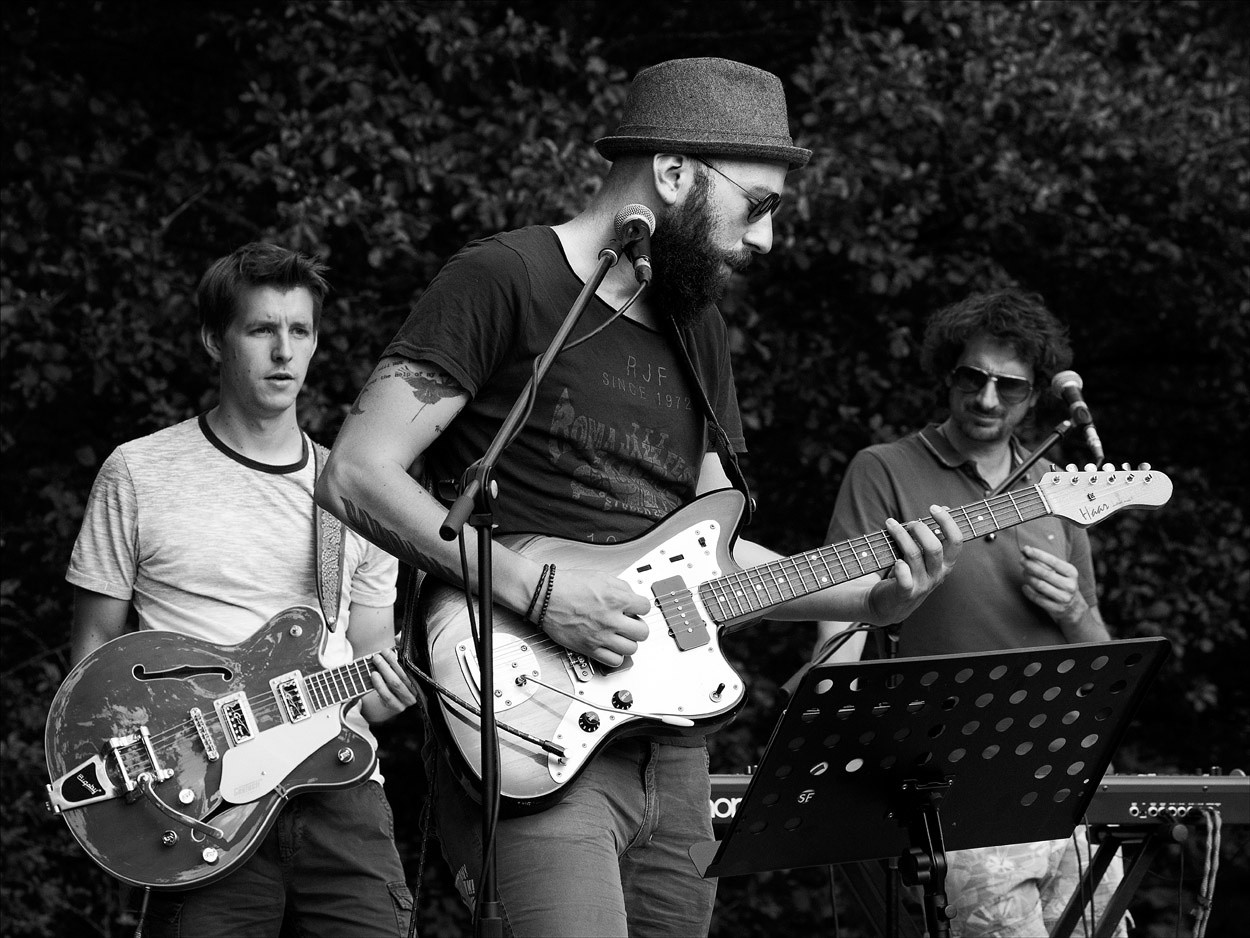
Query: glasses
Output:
[[948, 364, 1037, 407], [684, 151, 784, 224]]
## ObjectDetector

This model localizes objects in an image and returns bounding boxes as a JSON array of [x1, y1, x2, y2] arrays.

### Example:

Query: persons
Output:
[[65, 240, 418, 938], [811, 289, 1135, 938], [314, 58, 963, 938]]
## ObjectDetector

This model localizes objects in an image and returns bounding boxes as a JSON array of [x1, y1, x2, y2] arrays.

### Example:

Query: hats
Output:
[[593, 57, 812, 170]]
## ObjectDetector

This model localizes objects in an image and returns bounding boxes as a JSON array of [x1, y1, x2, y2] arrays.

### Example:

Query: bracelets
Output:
[[524, 564, 549, 621], [538, 564, 556, 629]]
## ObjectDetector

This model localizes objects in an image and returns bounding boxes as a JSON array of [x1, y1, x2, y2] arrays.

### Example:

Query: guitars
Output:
[[40, 597, 420, 886], [420, 461, 1173, 806]]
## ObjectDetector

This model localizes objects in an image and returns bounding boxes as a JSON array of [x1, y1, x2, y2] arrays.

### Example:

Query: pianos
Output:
[[704, 768, 1250, 937]]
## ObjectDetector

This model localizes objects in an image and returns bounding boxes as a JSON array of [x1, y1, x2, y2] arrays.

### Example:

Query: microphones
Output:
[[613, 204, 657, 286], [1050, 369, 1104, 461]]
